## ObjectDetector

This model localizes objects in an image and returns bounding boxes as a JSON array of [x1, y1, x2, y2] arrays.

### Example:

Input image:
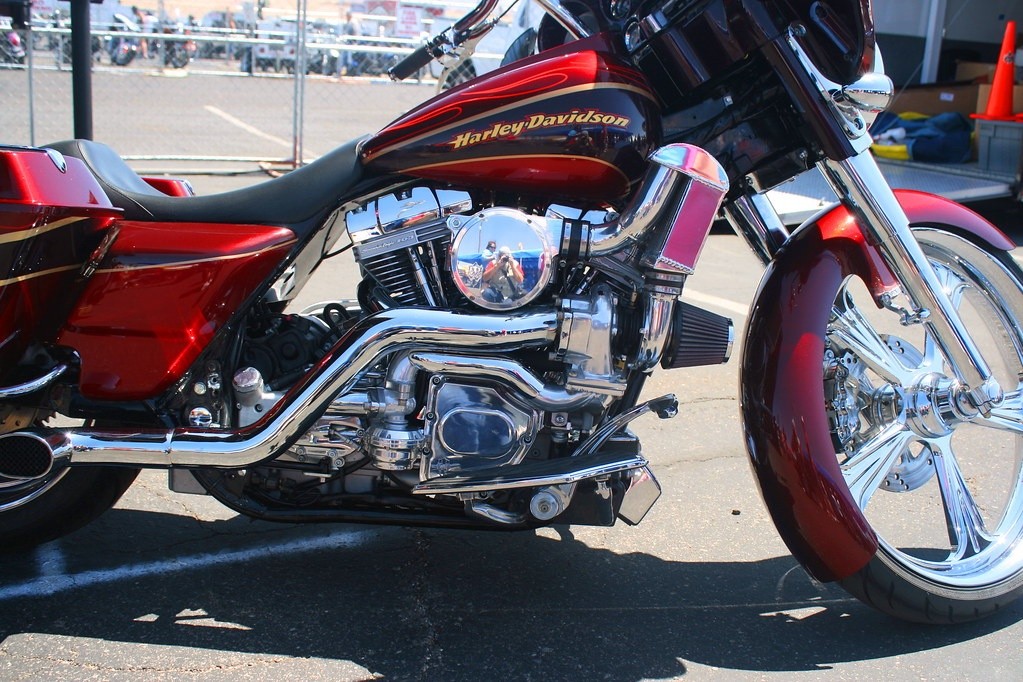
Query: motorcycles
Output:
[[1, 13, 428, 82], [0, 0, 1023, 629]]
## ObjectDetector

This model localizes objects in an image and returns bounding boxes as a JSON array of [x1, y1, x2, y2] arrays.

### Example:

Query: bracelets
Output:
[[495, 264, 499, 268]]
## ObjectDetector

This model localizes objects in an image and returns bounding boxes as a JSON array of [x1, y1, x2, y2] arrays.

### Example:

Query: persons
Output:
[[567, 126, 593, 156], [132, 7, 149, 60], [481, 241, 524, 302], [343, 12, 360, 71], [229, 13, 235, 64]]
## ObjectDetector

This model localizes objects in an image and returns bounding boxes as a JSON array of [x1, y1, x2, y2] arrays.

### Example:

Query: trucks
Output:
[[762, 1, 1023, 227]]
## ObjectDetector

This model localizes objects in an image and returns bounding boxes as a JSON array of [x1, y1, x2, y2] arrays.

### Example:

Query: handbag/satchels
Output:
[[510, 286, 526, 301]]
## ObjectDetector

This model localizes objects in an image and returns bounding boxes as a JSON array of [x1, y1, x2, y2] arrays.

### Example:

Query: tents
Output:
[[457, 248, 542, 292]]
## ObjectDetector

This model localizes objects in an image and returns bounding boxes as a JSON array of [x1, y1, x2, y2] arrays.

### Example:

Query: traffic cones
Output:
[[966, 17, 1023, 123]]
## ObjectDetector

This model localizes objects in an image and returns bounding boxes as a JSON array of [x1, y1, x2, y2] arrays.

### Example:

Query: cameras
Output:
[[503, 256, 510, 262]]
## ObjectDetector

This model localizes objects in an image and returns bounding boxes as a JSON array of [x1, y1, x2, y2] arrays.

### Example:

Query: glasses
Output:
[[489, 245, 496, 249]]
